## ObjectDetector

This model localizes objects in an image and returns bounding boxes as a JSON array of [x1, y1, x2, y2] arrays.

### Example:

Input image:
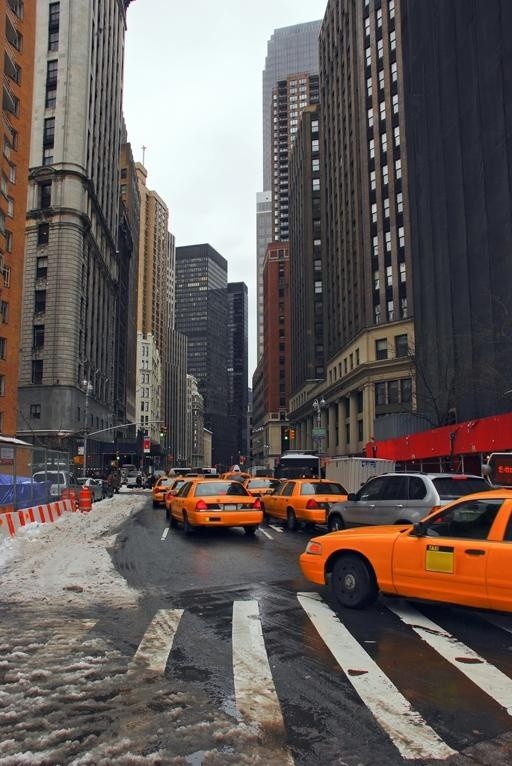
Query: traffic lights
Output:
[[159, 425, 164, 437], [163, 425, 169, 438], [284, 428, 289, 439], [291, 429, 296, 439]]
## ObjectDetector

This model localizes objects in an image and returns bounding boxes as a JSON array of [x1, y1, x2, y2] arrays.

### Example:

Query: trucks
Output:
[[318, 455, 398, 496]]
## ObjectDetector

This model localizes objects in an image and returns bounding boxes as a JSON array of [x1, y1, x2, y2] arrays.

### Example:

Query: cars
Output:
[[94, 478, 115, 499], [239, 472, 283, 513], [118, 460, 269, 534], [75, 476, 104, 504], [299, 483, 512, 620], [257, 474, 353, 531]]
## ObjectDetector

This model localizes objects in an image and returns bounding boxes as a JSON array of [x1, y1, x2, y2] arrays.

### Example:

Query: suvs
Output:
[[30, 469, 84, 507], [324, 467, 502, 536]]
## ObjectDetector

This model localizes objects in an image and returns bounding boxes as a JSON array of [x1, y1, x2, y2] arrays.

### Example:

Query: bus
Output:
[[269, 451, 323, 481]]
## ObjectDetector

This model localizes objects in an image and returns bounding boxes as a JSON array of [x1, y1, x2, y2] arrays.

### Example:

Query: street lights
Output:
[[250, 423, 274, 467], [310, 394, 328, 463], [238, 449, 241, 465], [79, 379, 95, 481], [230, 454, 233, 465]]
